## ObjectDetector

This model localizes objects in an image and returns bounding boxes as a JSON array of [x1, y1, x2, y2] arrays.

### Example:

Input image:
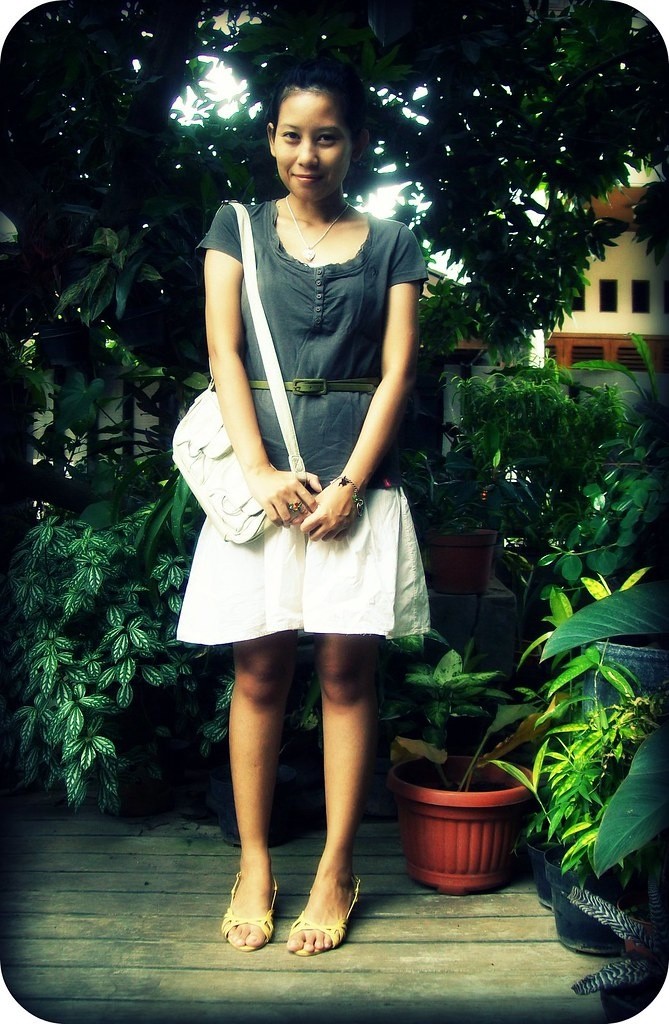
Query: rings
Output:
[[288, 501, 302, 513]]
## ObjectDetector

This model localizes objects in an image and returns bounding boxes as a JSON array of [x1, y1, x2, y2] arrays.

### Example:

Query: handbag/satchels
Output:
[[171, 388, 282, 545]]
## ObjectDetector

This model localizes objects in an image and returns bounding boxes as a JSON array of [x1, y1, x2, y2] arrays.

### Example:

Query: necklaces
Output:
[[285, 195, 350, 262]]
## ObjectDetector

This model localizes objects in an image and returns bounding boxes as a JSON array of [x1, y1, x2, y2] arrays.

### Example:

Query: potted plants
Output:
[[0, 333, 669, 1024]]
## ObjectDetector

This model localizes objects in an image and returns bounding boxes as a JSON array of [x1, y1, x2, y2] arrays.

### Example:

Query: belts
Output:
[[246, 379, 380, 398]]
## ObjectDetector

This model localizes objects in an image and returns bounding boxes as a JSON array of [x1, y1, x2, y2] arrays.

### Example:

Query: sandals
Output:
[[220, 867, 278, 953], [285, 873, 361, 957]]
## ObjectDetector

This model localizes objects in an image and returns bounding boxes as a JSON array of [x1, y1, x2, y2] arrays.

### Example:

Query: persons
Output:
[[176, 63, 432, 954]]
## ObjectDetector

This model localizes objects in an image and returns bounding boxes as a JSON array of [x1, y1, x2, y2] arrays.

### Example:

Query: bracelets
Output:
[[330, 476, 365, 517]]
